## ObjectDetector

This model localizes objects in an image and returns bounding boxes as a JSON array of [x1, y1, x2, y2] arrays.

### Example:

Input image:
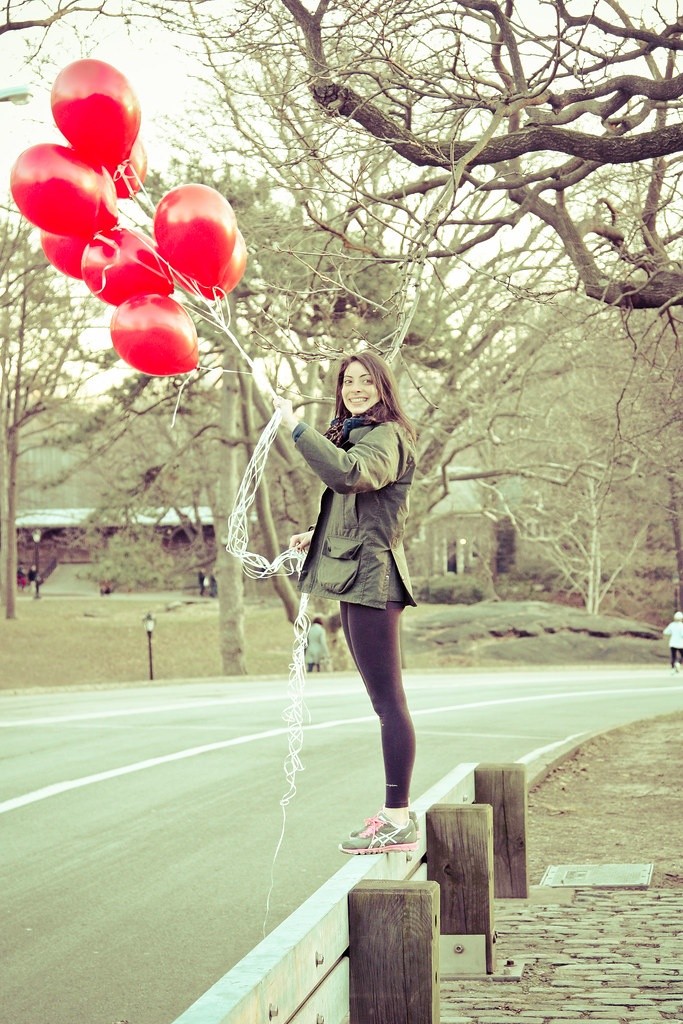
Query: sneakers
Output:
[[349, 809, 421, 840], [339, 811, 418, 854]]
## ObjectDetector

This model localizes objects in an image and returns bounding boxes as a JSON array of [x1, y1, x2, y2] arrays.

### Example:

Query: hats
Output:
[[673, 612, 682, 621]]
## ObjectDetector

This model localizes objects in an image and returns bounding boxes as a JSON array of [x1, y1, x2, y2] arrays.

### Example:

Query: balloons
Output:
[[12, 59, 248, 375]]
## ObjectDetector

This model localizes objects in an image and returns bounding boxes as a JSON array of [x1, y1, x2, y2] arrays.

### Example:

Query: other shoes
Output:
[[672, 668, 677, 674], [674, 662, 681, 673]]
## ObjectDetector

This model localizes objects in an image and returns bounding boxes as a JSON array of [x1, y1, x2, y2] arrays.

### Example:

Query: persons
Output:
[[273, 351, 424, 856], [198, 567, 217, 598], [18, 563, 37, 597], [302, 615, 329, 673], [663, 612, 683, 673]]
